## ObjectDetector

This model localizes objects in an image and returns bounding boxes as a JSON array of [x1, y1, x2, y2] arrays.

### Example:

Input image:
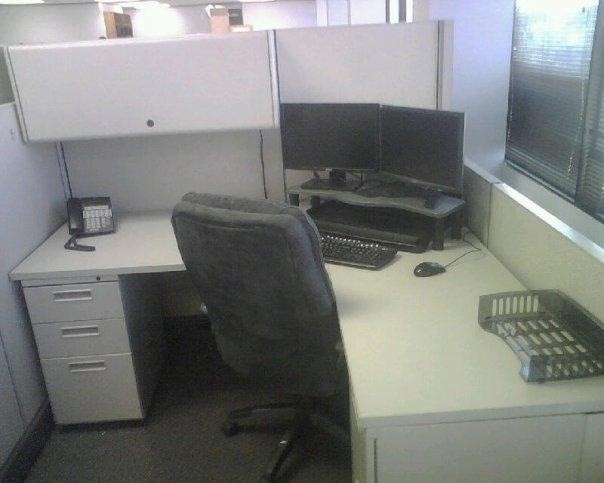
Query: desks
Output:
[[10, 210, 604, 483]]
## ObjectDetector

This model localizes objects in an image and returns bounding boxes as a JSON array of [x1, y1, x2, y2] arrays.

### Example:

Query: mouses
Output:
[[414, 261, 446, 277]]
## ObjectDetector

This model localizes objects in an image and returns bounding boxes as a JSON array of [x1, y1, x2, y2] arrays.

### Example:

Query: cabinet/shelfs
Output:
[[1, 29, 281, 144]]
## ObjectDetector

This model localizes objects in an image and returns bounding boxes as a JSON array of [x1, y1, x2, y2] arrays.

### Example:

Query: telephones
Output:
[[66, 196, 115, 235]]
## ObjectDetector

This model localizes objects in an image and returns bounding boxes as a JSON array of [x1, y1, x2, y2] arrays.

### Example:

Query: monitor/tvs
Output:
[[376, 104, 464, 198], [280, 102, 380, 190]]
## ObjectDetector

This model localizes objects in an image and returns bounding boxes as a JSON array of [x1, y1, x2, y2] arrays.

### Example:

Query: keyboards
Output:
[[319, 231, 398, 270]]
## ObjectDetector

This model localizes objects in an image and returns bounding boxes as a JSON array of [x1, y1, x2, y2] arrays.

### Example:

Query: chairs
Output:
[[167, 191, 348, 483]]
